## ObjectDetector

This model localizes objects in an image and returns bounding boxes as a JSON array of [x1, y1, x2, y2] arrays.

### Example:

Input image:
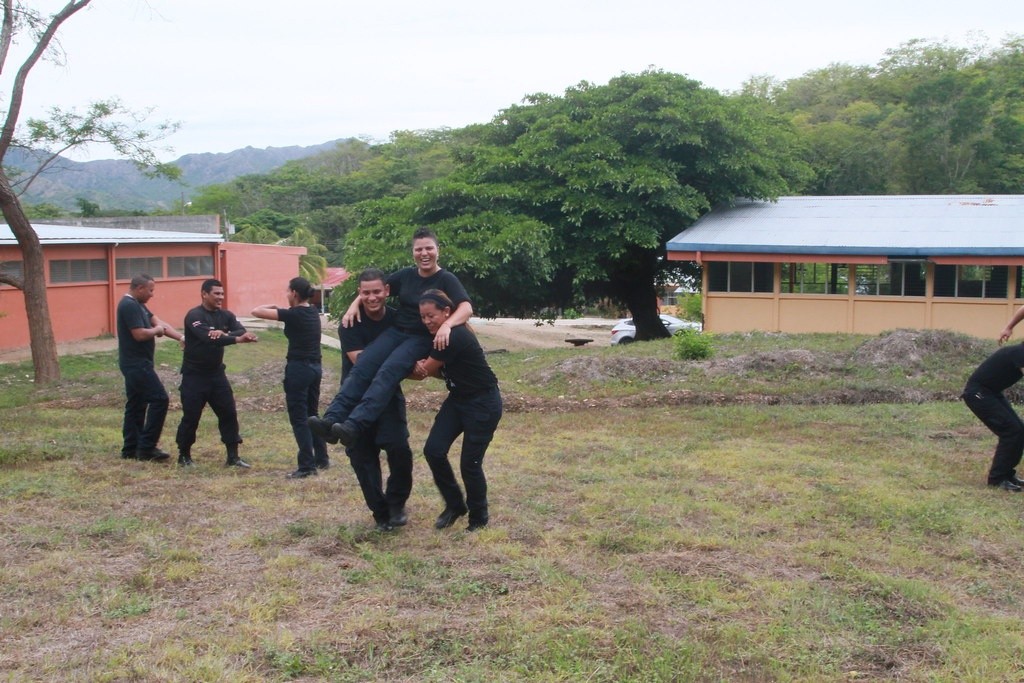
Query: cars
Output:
[[609, 314, 703, 347]]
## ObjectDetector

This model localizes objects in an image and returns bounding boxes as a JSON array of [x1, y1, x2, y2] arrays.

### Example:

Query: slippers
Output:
[[178, 448, 200, 468]]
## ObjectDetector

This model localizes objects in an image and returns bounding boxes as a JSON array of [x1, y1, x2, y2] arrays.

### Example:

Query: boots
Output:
[[226, 443, 252, 469]]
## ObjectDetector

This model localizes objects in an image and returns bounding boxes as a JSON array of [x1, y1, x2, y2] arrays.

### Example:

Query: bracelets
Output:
[[179, 334, 186, 344], [441, 322, 452, 329]]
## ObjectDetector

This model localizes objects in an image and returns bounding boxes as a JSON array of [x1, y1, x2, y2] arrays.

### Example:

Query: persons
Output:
[[116, 271, 185, 461], [307, 226, 502, 536], [176, 279, 258, 468], [252, 277, 329, 480], [959, 303, 1024, 493]]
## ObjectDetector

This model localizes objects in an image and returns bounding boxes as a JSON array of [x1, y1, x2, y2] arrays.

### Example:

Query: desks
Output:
[[565, 339, 593, 346]]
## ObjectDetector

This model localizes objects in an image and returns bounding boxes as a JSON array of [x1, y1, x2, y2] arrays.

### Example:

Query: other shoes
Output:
[[314, 462, 329, 469], [332, 423, 355, 446], [466, 520, 488, 532], [122, 448, 171, 460], [307, 416, 338, 444], [376, 510, 407, 532], [436, 508, 468, 529], [285, 469, 318, 479]]
